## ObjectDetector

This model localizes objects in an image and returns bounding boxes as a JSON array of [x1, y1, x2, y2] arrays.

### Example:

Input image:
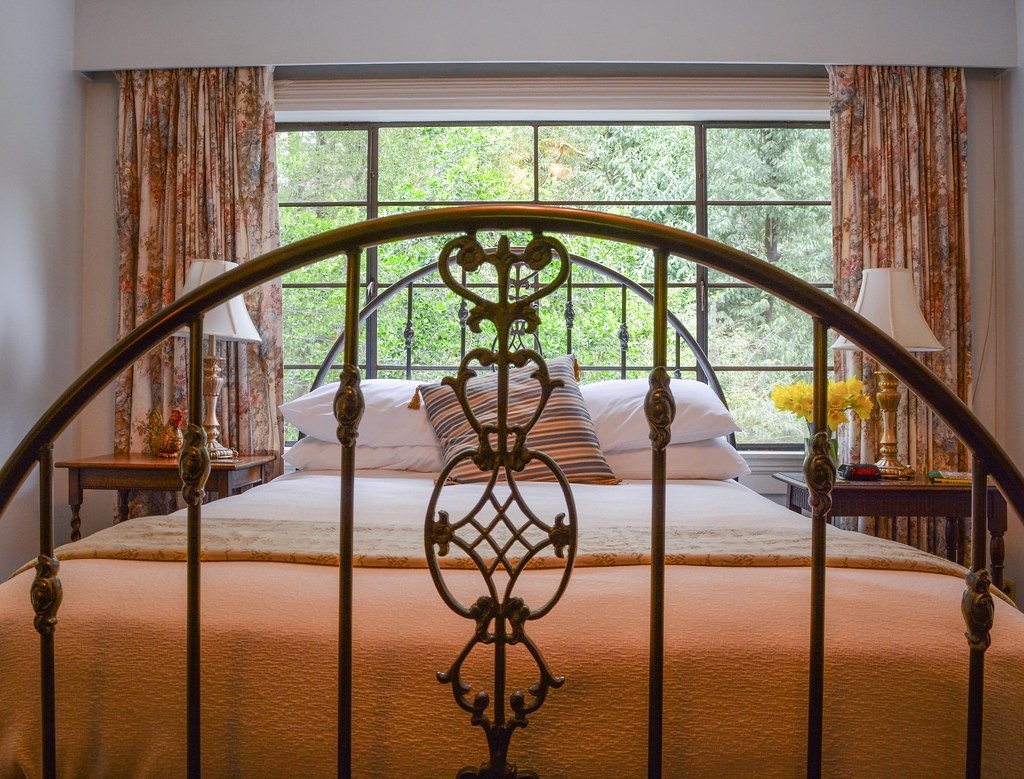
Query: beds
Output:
[[0, 468, 1023, 779]]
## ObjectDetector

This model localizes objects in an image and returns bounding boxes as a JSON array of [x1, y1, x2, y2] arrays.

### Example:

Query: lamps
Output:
[[831, 267, 949, 481], [169, 258, 263, 458]]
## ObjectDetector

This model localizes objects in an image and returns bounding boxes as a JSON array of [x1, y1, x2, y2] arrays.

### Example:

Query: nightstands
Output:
[[54, 450, 275, 544], [775, 470, 1009, 592]]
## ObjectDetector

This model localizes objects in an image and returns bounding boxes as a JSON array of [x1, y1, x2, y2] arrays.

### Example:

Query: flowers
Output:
[[774, 375, 872, 433]]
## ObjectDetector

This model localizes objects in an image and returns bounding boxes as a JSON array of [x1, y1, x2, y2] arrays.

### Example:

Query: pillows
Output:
[[599, 437, 753, 481], [579, 379, 744, 454], [281, 435, 445, 473], [276, 379, 441, 446], [408, 353, 624, 484]]
